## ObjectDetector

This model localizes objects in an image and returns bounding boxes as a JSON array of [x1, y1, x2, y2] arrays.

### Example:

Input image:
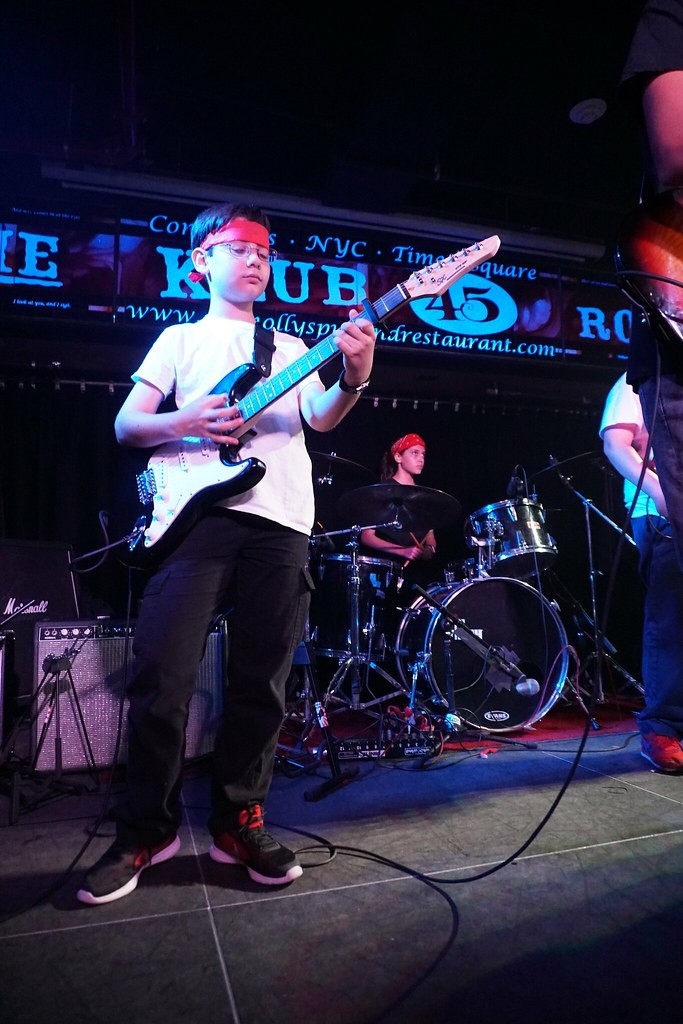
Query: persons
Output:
[[77, 203, 377, 905], [598, 371, 683, 772], [361, 433, 437, 639], [619, 0, 683, 567]]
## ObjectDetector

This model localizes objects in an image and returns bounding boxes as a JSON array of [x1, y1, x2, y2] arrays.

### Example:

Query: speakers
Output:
[[31, 619, 232, 774]]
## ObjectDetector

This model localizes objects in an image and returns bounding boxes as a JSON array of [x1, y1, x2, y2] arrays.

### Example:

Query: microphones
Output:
[[517, 679, 540, 697], [507, 467, 518, 498]]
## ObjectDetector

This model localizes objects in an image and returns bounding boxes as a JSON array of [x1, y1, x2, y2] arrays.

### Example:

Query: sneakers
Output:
[[209, 801, 304, 885], [76, 832, 181, 905], [640, 731, 683, 771]]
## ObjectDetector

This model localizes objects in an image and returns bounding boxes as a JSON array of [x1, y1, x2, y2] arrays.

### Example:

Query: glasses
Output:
[[211, 241, 278, 263]]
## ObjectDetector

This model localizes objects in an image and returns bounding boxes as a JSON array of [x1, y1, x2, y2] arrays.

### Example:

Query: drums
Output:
[[317, 554, 400, 665], [395, 577, 571, 732], [460, 498, 557, 576]]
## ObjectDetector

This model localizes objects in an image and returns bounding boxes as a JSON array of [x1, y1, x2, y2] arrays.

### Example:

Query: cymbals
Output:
[[311, 451, 366, 474], [342, 483, 459, 541]]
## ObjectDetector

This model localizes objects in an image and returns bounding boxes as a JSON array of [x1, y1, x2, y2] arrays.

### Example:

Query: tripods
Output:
[[409, 583, 538, 769], [280, 502, 444, 761], [553, 467, 648, 715]]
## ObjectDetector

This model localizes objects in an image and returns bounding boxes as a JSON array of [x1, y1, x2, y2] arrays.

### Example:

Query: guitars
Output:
[[106, 235, 502, 560], [612, 184, 683, 350]]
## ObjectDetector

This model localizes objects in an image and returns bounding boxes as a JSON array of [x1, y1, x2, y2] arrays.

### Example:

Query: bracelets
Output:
[[424, 544, 436, 553], [339, 369, 371, 394]]
